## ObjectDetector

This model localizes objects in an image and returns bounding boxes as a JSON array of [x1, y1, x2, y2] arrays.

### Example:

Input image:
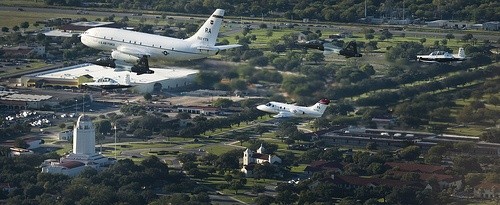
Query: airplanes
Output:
[[281, 39, 363, 61], [408, 46, 467, 67], [78, 74, 139, 95], [90, 53, 155, 76], [256, 98, 330, 118], [80, 9, 242, 64]]
